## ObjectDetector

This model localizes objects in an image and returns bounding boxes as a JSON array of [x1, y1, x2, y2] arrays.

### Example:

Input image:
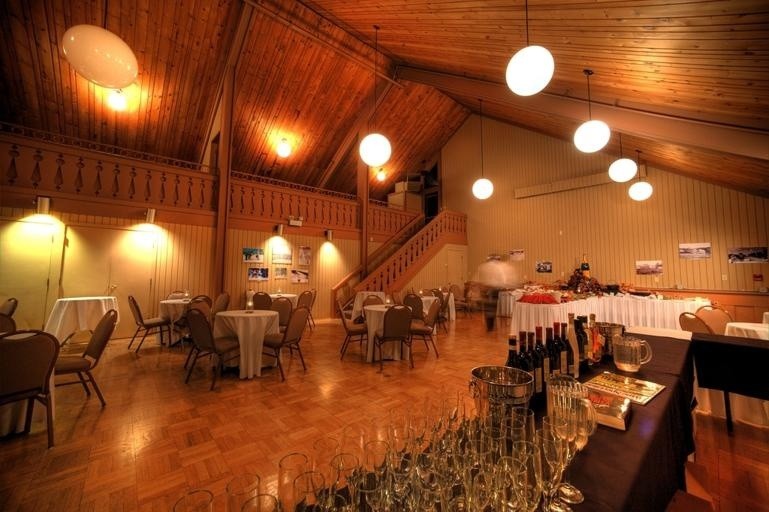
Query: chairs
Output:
[[262, 307, 310, 380], [440, 293, 451, 334], [0, 330, 60, 451], [54, 309, 118, 408], [430, 288, 445, 331], [210, 292, 231, 315], [410, 298, 441, 360], [127, 295, 172, 353], [311, 288, 317, 328], [186, 297, 213, 324], [372, 303, 415, 371], [693, 304, 733, 335], [297, 291, 314, 330], [338, 300, 368, 361], [304, 300, 306, 303], [362, 294, 383, 317], [253, 292, 272, 310], [403, 293, 423, 320], [271, 297, 293, 356], [184, 308, 239, 391], [1, 296, 18, 333], [679, 310, 713, 333], [446, 283, 471, 318]]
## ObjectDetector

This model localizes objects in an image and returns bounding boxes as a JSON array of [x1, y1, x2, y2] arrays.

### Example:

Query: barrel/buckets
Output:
[[467, 364, 535, 418]]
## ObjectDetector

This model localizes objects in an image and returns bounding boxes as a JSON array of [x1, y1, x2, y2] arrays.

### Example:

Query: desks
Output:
[[510, 295, 714, 342], [694, 322, 768, 431], [44, 296, 120, 345], [497, 290, 519, 317], [420, 295, 439, 318], [158, 300, 190, 327], [364, 304, 412, 362], [269, 294, 298, 311], [212, 307, 288, 386]]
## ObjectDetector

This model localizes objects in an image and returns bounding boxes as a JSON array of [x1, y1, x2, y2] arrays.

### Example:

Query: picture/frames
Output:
[[271, 247, 292, 264], [727, 246, 768, 264], [243, 247, 264, 263], [536, 261, 552, 272], [510, 249, 525, 260], [290, 269, 308, 283], [273, 267, 289, 279], [636, 260, 664, 275], [249, 267, 269, 281], [678, 242, 713, 260], [298, 246, 313, 266]]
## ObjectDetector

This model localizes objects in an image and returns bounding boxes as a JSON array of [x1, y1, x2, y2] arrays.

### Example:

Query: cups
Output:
[[240, 492, 280, 511], [172, 489, 213, 512], [278, 452, 309, 512], [225, 473, 260, 512], [547, 380, 597, 451], [612, 334, 653, 372], [293, 470, 324, 512], [311, 387, 585, 512]]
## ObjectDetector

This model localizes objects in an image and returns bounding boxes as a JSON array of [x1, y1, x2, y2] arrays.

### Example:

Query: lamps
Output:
[[628, 149, 654, 202], [378, 168, 385, 180], [276, 136, 291, 157], [471, 99, 494, 201], [607, 134, 638, 183], [572, 70, 611, 155], [58, 23, 141, 92], [38, 197, 50, 214], [505, 1, 556, 98], [146, 208, 156, 223], [327, 230, 332, 240], [109, 89, 127, 111], [278, 224, 283, 236], [360, 24, 392, 170]]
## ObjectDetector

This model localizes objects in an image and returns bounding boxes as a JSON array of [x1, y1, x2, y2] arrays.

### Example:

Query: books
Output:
[[573, 386, 632, 431], [585, 371, 666, 404]]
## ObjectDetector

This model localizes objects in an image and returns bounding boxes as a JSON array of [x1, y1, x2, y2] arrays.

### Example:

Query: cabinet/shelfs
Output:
[[292, 331, 697, 510]]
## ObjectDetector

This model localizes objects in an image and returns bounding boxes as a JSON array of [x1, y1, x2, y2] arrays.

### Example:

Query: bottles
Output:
[[589, 314, 602, 364], [518, 332, 535, 409], [575, 320, 584, 365], [536, 326, 550, 394], [568, 313, 580, 379], [581, 254, 590, 278], [561, 323, 575, 380], [546, 327, 564, 376], [504, 335, 522, 370], [528, 332, 547, 407], [577, 316, 594, 366], [554, 323, 568, 376]]
